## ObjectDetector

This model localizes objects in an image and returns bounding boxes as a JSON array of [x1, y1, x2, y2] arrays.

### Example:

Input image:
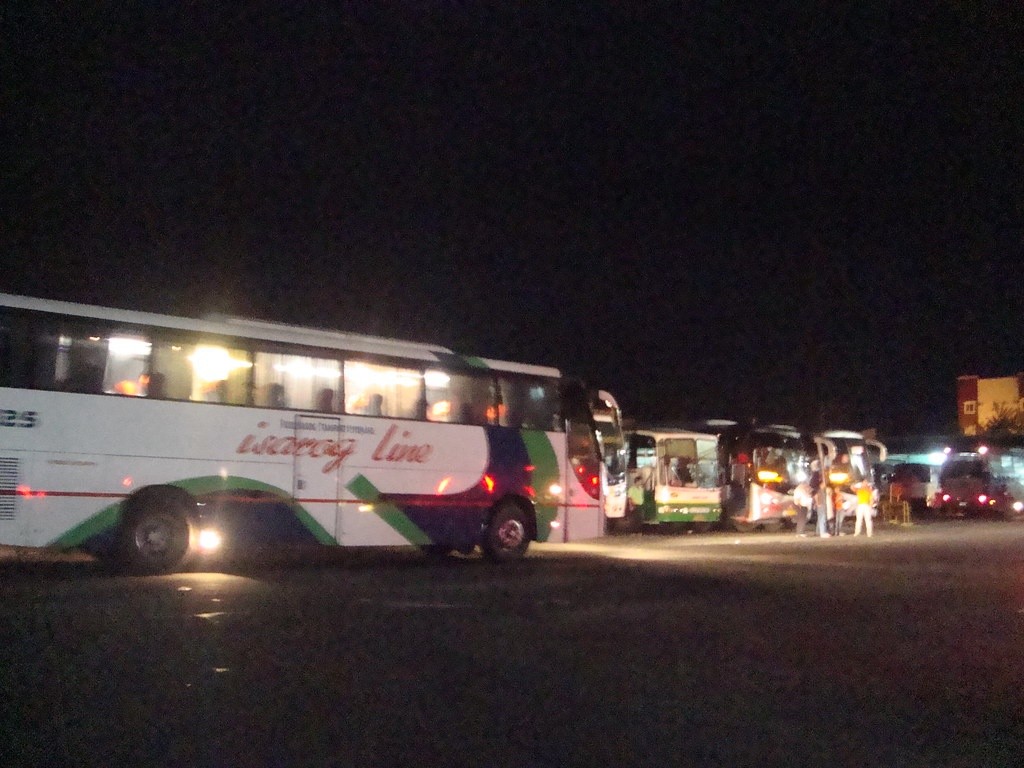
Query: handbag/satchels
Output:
[[841, 500, 850, 511]]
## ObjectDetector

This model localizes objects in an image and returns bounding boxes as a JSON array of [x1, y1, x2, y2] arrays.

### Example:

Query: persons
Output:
[[668, 473, 682, 487], [104, 366, 151, 397], [627, 476, 644, 537], [850, 479, 874, 537], [792, 482, 844, 540]]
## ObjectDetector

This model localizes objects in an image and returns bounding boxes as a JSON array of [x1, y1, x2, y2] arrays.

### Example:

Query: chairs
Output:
[[361, 394, 386, 416], [448, 401, 472, 423], [311, 389, 335, 412], [259, 382, 286, 408], [145, 372, 165, 397], [405, 400, 428, 420]]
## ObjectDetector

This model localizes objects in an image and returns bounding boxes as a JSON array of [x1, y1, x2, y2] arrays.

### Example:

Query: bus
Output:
[[0, 294, 606, 576], [700, 418, 1024, 520], [625, 426, 720, 531], [596, 391, 629, 522]]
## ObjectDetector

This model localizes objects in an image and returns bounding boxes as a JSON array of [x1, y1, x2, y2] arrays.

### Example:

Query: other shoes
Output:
[[820, 533, 831, 538], [796, 534, 807, 538]]
